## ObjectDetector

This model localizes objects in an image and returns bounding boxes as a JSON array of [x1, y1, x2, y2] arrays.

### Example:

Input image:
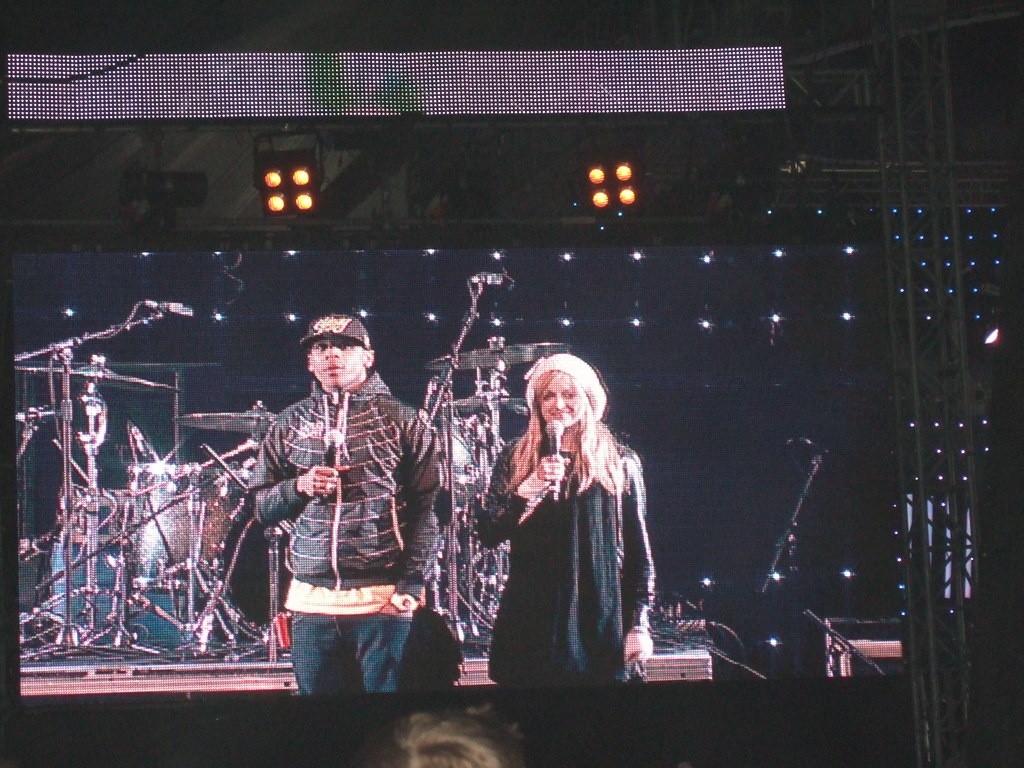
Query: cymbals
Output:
[[15, 363, 183, 398], [422, 341, 572, 374], [439, 394, 527, 414], [172, 409, 279, 433]]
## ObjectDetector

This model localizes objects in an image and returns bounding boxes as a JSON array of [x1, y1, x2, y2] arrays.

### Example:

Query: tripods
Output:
[[424, 284, 506, 642], [15, 350, 270, 659]]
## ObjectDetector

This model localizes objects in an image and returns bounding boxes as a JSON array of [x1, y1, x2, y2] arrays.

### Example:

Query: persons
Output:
[[248, 313, 444, 693], [351, 705, 527, 768], [473, 352, 658, 692]]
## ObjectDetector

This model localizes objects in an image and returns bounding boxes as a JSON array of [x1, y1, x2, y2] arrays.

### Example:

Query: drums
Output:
[[128, 461, 236, 588], [434, 423, 511, 634]]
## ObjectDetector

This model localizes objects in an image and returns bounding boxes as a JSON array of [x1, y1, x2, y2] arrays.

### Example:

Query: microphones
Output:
[[545, 418, 564, 501], [319, 429, 343, 500], [470, 271, 516, 291]]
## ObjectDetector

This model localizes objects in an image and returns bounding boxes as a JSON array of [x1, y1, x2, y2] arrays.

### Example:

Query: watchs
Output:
[[391, 594, 419, 612]]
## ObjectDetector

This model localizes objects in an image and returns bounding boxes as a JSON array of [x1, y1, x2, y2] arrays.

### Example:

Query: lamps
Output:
[[252, 125, 322, 217], [584, 156, 642, 214]]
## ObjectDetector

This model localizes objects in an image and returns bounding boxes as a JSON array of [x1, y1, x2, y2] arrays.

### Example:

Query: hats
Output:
[[301, 315, 372, 350], [526, 353, 608, 421]]
